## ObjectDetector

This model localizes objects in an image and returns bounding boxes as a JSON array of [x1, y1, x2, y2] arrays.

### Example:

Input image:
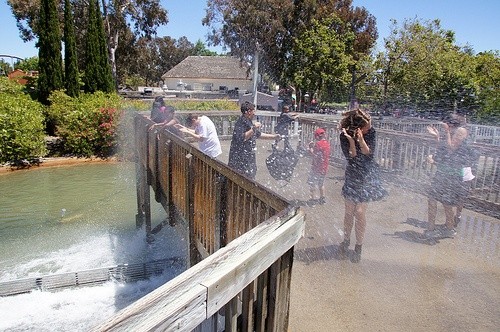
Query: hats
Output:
[[315, 129, 325, 139]]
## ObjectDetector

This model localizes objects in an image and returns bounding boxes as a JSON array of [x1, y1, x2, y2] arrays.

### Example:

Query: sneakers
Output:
[[414, 229, 441, 240], [435, 226, 457, 239]]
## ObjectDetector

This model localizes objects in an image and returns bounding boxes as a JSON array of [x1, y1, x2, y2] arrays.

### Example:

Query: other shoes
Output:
[[318, 199, 326, 205], [454, 215, 462, 223], [351, 251, 361, 263], [337, 242, 349, 254], [307, 200, 317, 207]]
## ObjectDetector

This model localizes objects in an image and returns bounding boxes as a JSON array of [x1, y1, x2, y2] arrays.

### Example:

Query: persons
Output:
[[367, 100, 401, 120], [416, 113, 478, 242], [340, 108, 389, 263], [175, 113, 223, 159], [304, 129, 330, 205], [150, 95, 166, 122], [271, 106, 299, 151], [227, 101, 282, 180], [148, 106, 185, 139], [299, 100, 339, 115]]
